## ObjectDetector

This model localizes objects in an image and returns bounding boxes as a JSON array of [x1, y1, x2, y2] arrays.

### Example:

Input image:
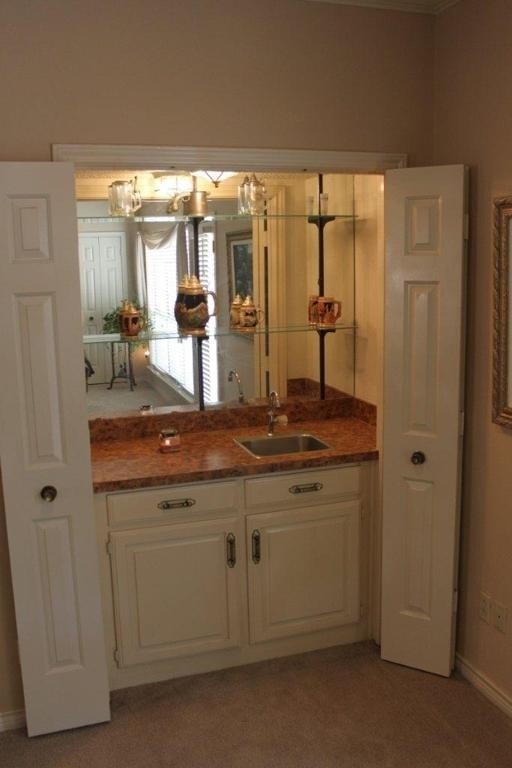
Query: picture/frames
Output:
[[224, 227, 255, 341]]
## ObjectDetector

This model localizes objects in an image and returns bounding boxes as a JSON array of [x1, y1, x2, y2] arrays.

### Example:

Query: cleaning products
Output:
[[138, 402, 155, 416]]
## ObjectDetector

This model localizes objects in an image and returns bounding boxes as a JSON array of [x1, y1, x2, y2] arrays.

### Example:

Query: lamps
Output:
[[150, 169, 190, 198], [189, 169, 240, 187]]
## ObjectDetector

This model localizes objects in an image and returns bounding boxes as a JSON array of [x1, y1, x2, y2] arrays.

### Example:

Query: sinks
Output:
[[233, 432, 335, 460]]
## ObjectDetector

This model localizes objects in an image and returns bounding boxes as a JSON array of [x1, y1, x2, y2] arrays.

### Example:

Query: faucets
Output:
[[267, 390, 281, 437], [227, 369, 244, 403]]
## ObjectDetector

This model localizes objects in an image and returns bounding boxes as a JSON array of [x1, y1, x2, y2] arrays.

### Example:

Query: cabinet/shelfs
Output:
[[90, 458, 376, 691]]
